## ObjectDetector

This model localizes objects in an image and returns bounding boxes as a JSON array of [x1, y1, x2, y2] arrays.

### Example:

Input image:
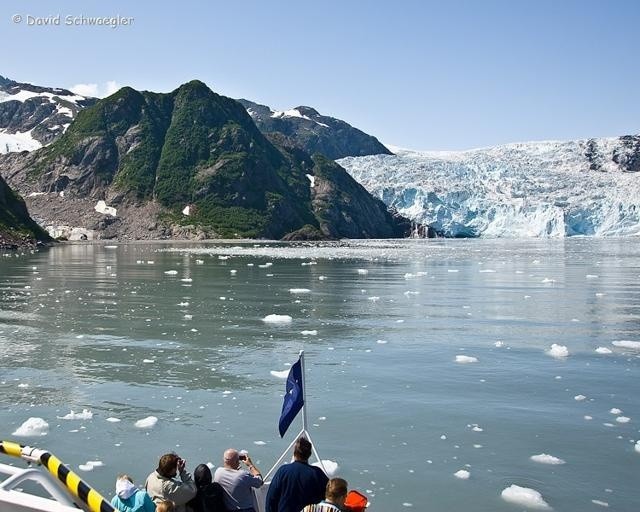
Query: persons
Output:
[[145, 454, 197, 512], [188, 464, 228, 512], [265, 437, 329, 511], [110, 475, 157, 512], [301, 477, 348, 512], [214, 449, 264, 512], [154, 501, 174, 512]]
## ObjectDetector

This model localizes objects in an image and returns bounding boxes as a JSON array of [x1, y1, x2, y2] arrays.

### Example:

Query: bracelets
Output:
[[249, 465, 254, 470]]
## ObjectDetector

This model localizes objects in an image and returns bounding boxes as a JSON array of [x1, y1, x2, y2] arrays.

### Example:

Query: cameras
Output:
[[177, 457, 183, 465], [238, 455, 246, 461]]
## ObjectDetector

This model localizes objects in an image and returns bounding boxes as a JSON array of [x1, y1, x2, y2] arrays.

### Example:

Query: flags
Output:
[[278, 359, 304, 437]]
